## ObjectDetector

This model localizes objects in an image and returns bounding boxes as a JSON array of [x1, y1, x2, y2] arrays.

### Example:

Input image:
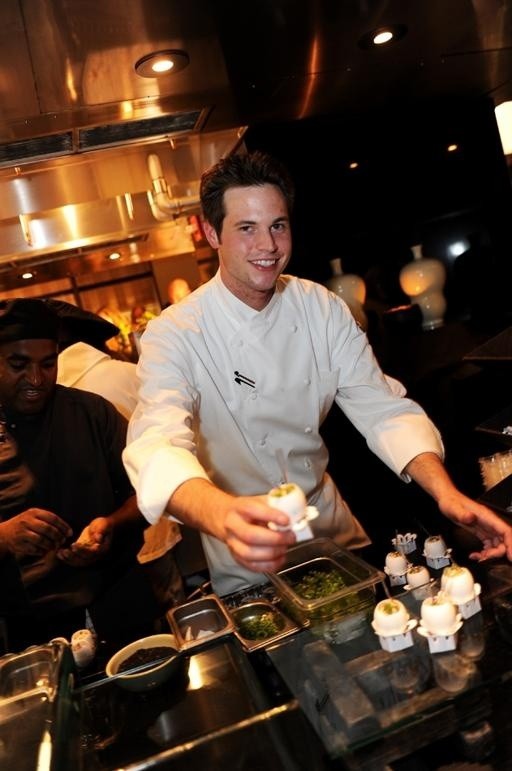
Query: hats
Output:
[[0, 299, 120, 345]]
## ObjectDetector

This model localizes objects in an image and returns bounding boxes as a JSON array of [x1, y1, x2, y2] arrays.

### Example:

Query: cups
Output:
[[477, 449, 512, 493]]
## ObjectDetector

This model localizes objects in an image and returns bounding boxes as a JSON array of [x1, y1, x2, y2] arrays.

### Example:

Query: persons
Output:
[[53, 328, 188, 606], [118, 147, 511, 599], [2, 294, 156, 647]]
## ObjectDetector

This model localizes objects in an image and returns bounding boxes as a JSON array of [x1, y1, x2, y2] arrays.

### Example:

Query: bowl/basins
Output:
[[104, 634, 176, 692]]
